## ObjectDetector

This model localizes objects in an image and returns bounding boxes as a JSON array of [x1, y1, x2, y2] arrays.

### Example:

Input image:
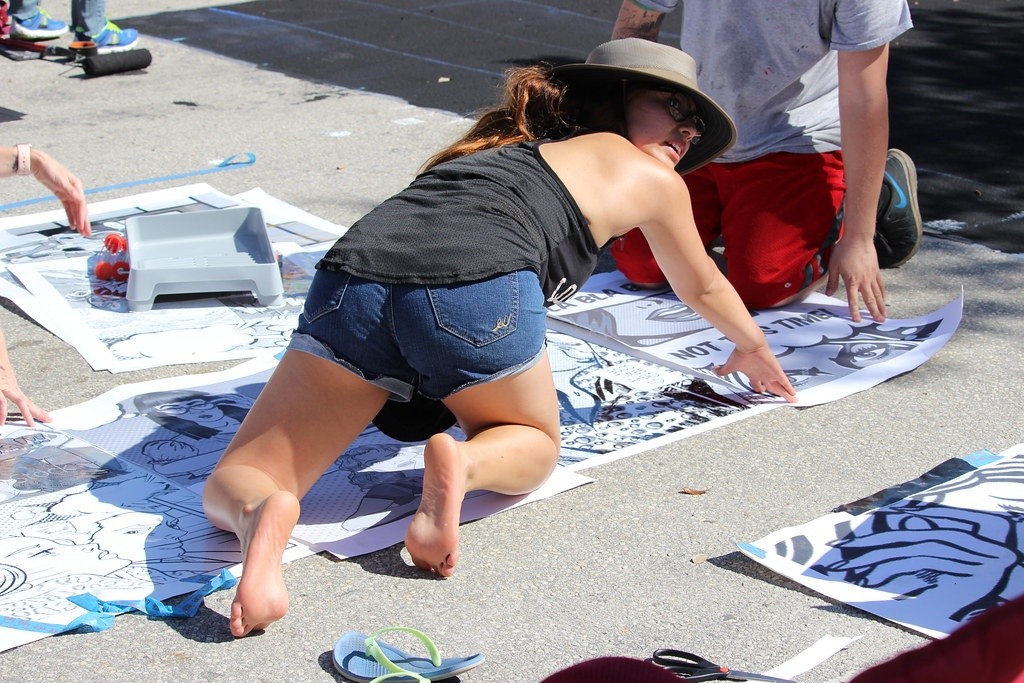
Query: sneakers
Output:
[[73, 20, 139, 54], [873, 148, 921, 268], [9, 8, 69, 39]]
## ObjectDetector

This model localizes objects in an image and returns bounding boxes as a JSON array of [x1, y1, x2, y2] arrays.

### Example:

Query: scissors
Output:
[[652, 647, 795, 683]]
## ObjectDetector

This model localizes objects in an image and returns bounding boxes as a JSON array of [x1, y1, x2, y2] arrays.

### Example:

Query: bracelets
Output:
[[13, 143, 33, 175]]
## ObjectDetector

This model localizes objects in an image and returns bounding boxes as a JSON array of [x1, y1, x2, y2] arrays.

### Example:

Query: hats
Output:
[[543, 36, 737, 178]]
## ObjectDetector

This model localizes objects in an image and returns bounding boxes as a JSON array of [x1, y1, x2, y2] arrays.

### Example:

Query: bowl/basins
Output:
[[69, 41, 99, 57]]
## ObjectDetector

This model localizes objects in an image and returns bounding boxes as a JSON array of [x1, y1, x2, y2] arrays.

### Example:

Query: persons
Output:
[[204, 39, 795, 641], [609, 1, 924, 326], [6, 0, 139, 54], [0, 142, 92, 240]]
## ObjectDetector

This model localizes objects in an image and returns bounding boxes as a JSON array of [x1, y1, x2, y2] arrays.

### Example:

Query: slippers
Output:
[[331, 626, 485, 683]]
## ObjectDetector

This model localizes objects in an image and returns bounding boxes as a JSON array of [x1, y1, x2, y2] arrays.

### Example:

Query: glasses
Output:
[[641, 84, 707, 144]]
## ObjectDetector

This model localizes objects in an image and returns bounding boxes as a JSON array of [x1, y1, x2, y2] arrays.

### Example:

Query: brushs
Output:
[[0, 38, 152, 76]]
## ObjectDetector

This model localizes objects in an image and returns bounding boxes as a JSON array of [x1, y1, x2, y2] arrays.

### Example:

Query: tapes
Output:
[[68, 41, 98, 58]]
